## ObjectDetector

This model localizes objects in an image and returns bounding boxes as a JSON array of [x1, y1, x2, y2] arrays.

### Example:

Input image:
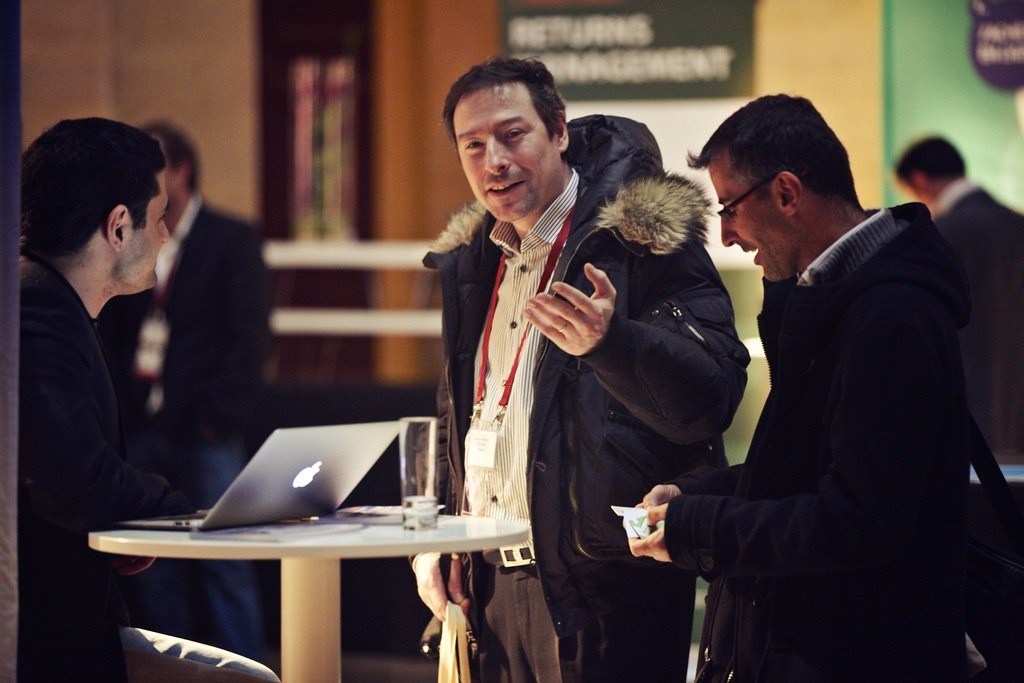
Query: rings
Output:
[[558, 322, 569, 331]]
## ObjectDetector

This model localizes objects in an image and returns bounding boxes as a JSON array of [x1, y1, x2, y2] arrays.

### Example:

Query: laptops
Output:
[[116, 420, 409, 531]]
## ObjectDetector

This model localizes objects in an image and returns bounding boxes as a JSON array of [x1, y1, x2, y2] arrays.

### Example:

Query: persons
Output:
[[98, 124, 273, 658], [896, 135, 1024, 453], [627, 94, 985, 683], [406, 57, 751, 683], [18, 118, 281, 683]]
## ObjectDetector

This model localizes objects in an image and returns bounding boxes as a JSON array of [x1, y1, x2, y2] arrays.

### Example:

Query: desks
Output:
[[87, 512, 534, 683]]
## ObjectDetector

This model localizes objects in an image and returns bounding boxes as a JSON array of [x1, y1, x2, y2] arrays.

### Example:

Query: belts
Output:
[[480, 543, 536, 569]]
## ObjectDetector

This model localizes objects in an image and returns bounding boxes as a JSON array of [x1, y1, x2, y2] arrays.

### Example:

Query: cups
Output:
[[400, 417, 439, 529]]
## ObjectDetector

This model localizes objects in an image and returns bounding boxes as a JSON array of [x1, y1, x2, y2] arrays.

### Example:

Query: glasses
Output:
[[717, 165, 801, 226]]
